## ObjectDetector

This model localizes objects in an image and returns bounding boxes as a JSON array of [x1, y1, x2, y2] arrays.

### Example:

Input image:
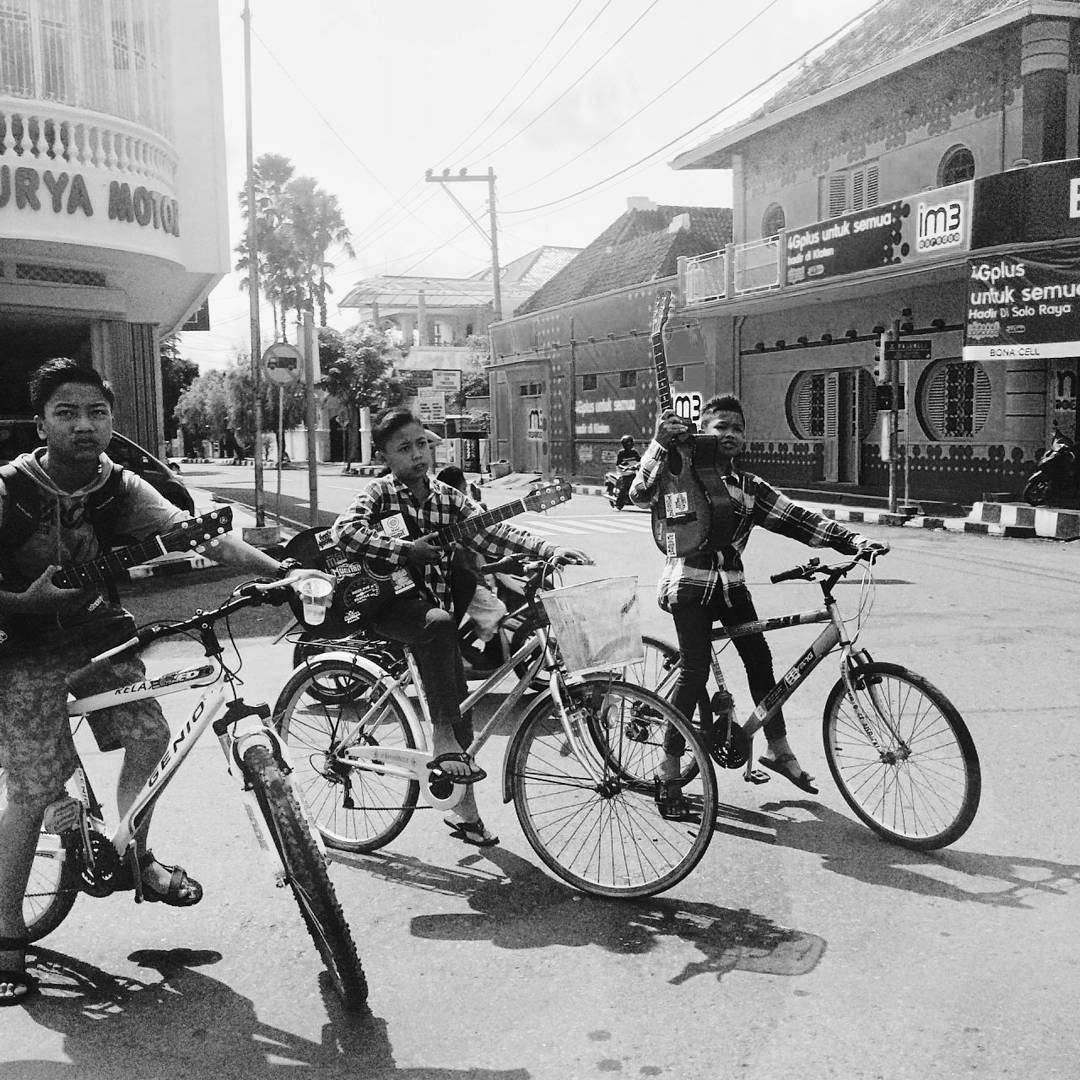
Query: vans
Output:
[[0, 418, 195, 549]]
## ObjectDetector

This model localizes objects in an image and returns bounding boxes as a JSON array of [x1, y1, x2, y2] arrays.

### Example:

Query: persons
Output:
[[0, 358, 336, 1005], [613, 436, 641, 511], [331, 407, 598, 846], [438, 463, 506, 643], [630, 395, 891, 822], [1039, 429, 1078, 509], [275, 453, 289, 469]]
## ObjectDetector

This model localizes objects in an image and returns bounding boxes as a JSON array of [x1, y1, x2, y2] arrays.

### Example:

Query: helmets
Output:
[[621, 436, 634, 450]]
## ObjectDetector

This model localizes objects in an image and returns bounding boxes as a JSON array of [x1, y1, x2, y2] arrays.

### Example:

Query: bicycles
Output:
[[0, 574, 372, 1011], [269, 551, 720, 901], [583, 544, 981, 851]]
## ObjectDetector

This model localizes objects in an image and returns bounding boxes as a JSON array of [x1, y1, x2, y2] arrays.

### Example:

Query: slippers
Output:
[[444, 816, 498, 846], [759, 755, 818, 794], [426, 752, 487, 783], [656, 776, 687, 820]]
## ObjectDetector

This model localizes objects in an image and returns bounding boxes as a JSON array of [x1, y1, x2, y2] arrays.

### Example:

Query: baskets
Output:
[[537, 575, 645, 675]]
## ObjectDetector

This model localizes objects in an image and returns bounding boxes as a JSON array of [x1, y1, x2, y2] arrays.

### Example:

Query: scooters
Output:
[[1022, 420, 1080, 506], [271, 548, 568, 705], [602, 463, 641, 512]]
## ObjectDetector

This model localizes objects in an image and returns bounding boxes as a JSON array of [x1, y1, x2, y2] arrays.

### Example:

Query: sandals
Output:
[[123, 851, 203, 906], [0, 969, 29, 1007]]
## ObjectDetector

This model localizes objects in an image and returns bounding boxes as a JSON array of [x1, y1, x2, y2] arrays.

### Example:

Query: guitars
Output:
[[647, 289, 734, 559], [276, 477, 573, 641], [0, 504, 236, 658]]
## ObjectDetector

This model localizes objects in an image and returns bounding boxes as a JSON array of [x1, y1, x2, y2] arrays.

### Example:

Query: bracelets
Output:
[[276, 557, 302, 582]]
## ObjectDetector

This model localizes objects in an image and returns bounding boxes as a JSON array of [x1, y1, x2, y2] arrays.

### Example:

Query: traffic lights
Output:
[[872, 333, 892, 385]]
